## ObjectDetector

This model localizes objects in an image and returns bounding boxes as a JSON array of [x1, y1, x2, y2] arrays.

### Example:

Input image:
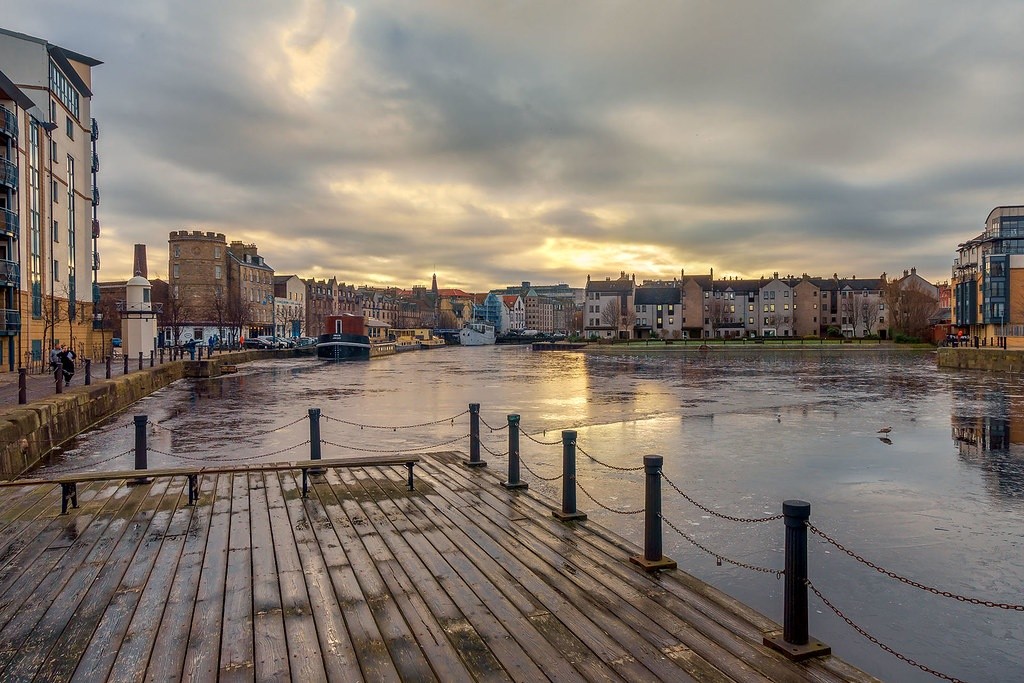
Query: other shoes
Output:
[[66, 382, 69, 387]]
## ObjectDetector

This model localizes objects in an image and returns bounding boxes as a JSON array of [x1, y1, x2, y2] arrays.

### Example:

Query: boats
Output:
[[316, 313, 397, 361], [459, 322, 497, 346], [387, 328, 446, 351]]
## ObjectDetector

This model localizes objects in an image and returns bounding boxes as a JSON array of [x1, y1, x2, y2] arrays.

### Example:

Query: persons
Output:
[[178, 334, 247, 360], [957, 330, 962, 343], [49, 344, 77, 387]]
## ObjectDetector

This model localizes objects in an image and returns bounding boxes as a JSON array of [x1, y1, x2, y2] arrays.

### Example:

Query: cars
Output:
[[241, 335, 318, 349], [113, 338, 123, 347]]
